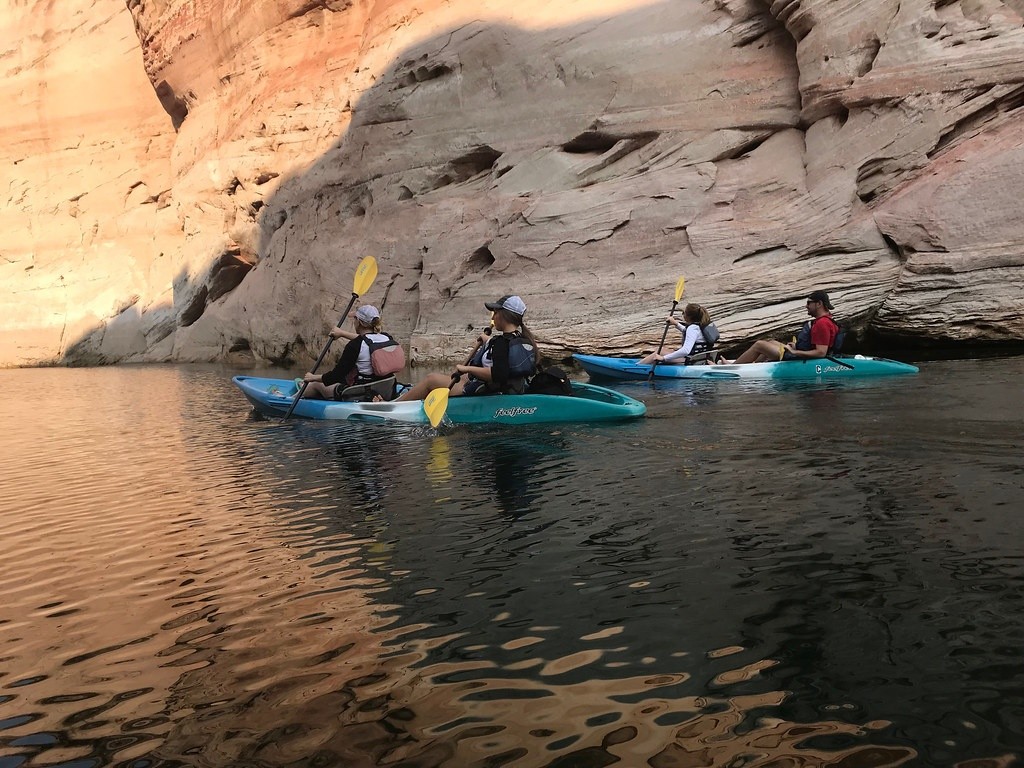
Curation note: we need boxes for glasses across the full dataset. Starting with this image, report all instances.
[807,301,817,305]
[682,311,687,316]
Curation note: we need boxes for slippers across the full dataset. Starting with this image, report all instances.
[372,395,386,402]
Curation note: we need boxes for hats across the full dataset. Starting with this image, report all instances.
[485,295,527,316]
[809,290,834,310]
[348,305,380,324]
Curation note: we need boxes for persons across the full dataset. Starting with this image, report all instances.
[634,304,710,364]
[707,290,838,364]
[371,295,539,401]
[267,305,395,400]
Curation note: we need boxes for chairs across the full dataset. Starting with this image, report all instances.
[341,373,396,402]
[684,350,719,365]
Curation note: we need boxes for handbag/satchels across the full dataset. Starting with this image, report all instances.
[531,366,575,396]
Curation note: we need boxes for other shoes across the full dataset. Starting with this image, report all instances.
[268,388,280,395]
[295,378,303,391]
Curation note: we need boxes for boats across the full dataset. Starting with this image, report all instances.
[571,353,921,381]
[231,375,649,425]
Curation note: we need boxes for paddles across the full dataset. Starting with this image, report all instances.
[648,276,685,382]
[779,336,797,361]
[284,255,378,421]
[423,310,497,428]
